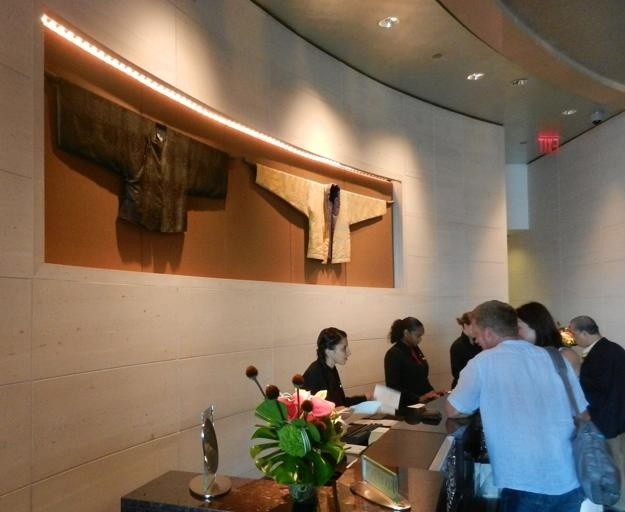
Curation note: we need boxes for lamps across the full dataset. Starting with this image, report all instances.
[469,72,482,80]
[380,17,398,28]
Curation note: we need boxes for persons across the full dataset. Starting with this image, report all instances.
[450,312,484,391]
[302,327,374,412]
[443,300,590,512]
[515,302,584,378]
[568,315,625,512]
[384,317,451,404]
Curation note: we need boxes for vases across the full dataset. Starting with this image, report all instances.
[289,462,315,503]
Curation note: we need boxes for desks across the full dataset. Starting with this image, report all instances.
[393,392,476,508]
[122,429,456,512]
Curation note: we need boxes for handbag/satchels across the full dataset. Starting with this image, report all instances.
[546,344,621,506]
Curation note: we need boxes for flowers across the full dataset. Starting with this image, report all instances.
[245,366,346,487]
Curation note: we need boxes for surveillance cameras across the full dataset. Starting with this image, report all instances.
[590,111,603,126]
